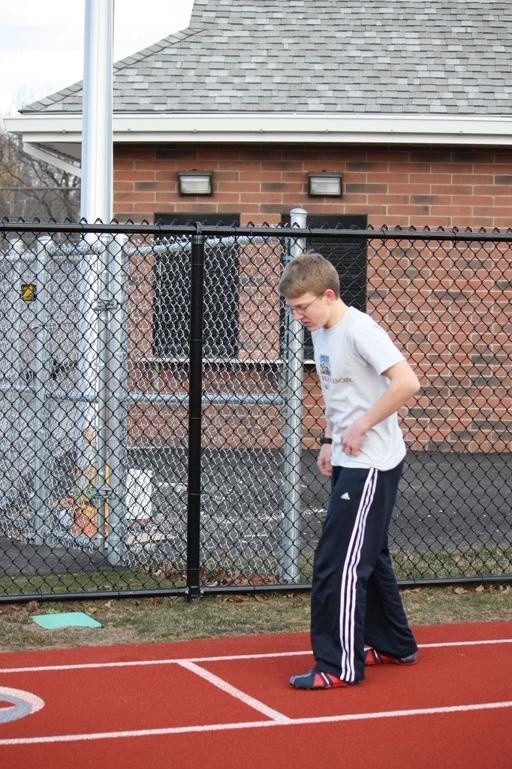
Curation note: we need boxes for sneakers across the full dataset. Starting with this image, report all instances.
[363,648,417,666]
[288,669,359,690]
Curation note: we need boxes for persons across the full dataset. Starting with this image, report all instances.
[277,248,422,692]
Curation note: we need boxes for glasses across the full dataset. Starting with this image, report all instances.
[285,296,319,315]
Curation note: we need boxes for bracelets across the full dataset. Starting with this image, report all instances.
[318,437,331,446]
